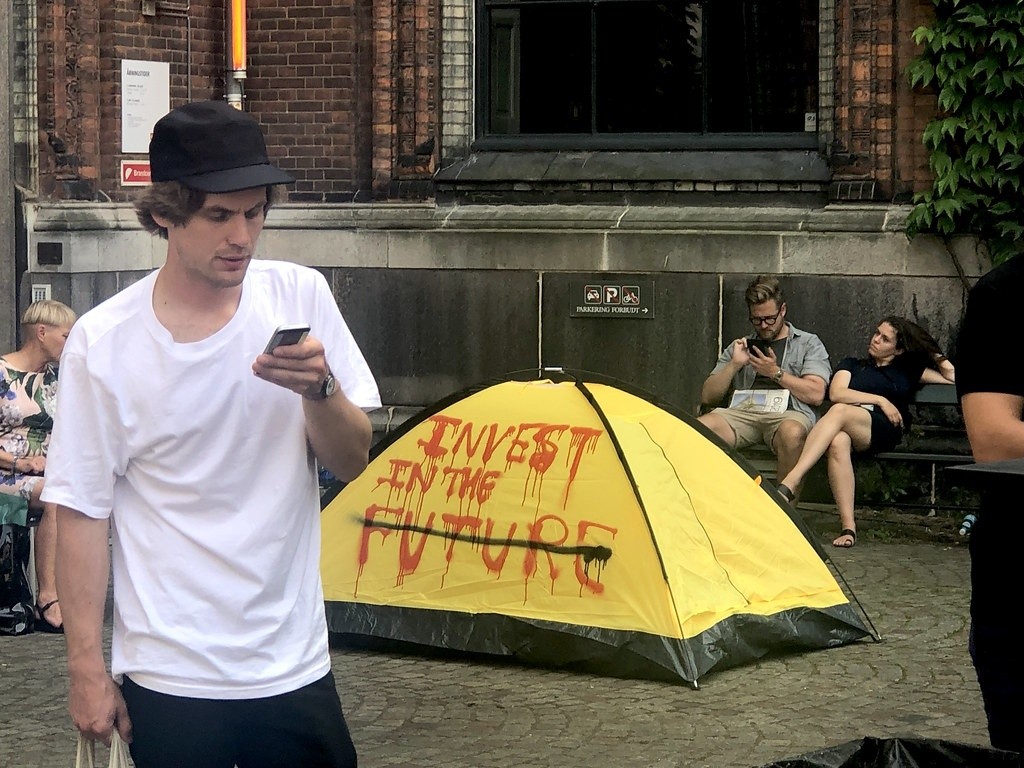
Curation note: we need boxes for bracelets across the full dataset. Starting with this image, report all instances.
[772,369,785,383]
[937,356,947,364]
[11,456,18,472]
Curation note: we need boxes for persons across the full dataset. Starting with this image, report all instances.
[697,278,832,508]
[776,316,956,547]
[0,300,75,635]
[38,101,381,768]
[950,251,1023,752]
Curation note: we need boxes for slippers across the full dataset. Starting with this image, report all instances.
[833,529,856,548]
[778,482,796,501]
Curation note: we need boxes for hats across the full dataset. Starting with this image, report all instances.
[149,100,296,194]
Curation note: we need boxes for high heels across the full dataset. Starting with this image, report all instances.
[34,599,63,633]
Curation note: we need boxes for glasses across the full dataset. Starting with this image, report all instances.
[749,308,781,325]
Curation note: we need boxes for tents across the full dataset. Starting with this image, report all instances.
[316,366,880,684]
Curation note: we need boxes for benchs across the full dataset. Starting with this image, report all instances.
[693,381,973,517]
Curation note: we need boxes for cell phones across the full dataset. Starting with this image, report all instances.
[746,338,766,359]
[253,322,311,376]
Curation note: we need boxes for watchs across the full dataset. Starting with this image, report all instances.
[301,371,335,400]
